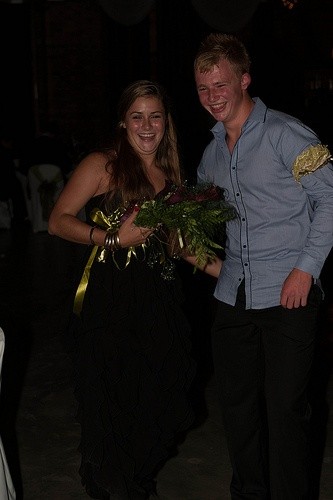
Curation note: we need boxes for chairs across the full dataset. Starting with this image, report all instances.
[0,164,86,232]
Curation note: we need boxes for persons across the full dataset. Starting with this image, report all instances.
[47,79,189,500]
[194,33,333,500]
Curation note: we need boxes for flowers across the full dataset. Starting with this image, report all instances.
[107,179,240,282]
